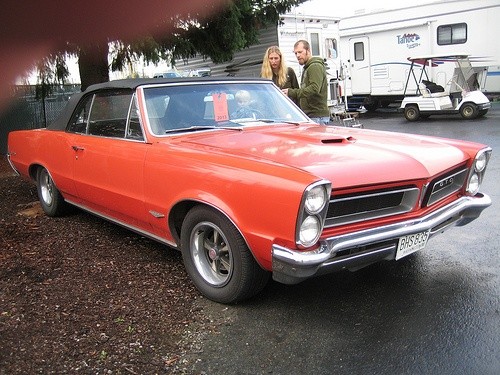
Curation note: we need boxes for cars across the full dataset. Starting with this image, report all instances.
[7,76,494,306]
[399,52,491,122]
[154,72,183,78]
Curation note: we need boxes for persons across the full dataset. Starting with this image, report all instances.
[230,90,262,125]
[257,45,301,115]
[279,38,331,126]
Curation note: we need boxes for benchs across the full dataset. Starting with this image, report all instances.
[419,82,450,98]
